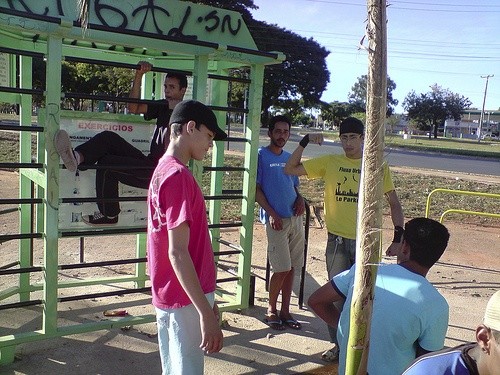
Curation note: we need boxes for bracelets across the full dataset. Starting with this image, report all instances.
[299,134,310,149]
[392,226,405,243]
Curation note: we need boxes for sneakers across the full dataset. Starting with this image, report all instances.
[82,211,119,227]
[54,129,77,172]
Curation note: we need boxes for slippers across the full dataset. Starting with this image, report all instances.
[285,314,302,329]
[263,317,285,330]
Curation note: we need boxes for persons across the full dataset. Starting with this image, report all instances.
[147,100,227,375]
[284,117,404,362]
[256,115,305,331]
[401,290,500,375]
[307,217,450,375]
[54,60,188,227]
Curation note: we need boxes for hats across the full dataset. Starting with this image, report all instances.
[168,99,227,141]
[483,290,499,331]
[338,117,364,135]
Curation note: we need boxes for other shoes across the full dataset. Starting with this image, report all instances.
[321,344,339,362]
